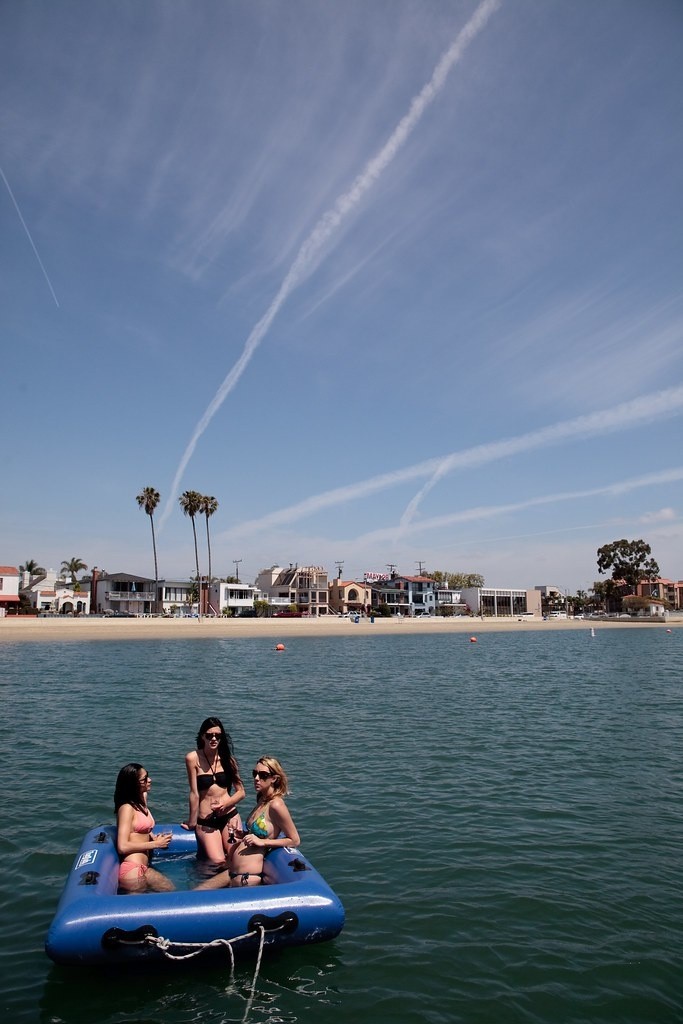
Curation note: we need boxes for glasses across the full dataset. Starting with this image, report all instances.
[139,771,149,784]
[204,733,222,740]
[252,769,272,779]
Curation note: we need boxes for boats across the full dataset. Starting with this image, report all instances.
[45,824,346,974]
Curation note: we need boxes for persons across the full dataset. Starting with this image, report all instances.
[190,755,300,891]
[180,716,246,875]
[114,762,176,894]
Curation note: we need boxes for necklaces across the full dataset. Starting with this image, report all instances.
[203,749,219,780]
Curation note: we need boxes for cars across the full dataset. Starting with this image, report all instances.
[185,613,223,618]
[517,611,633,619]
[415,611,431,618]
[271,609,302,618]
[230,610,258,618]
[342,611,359,618]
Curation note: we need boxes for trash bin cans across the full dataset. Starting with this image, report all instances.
[371,616,374,623]
[355,616,358,623]
